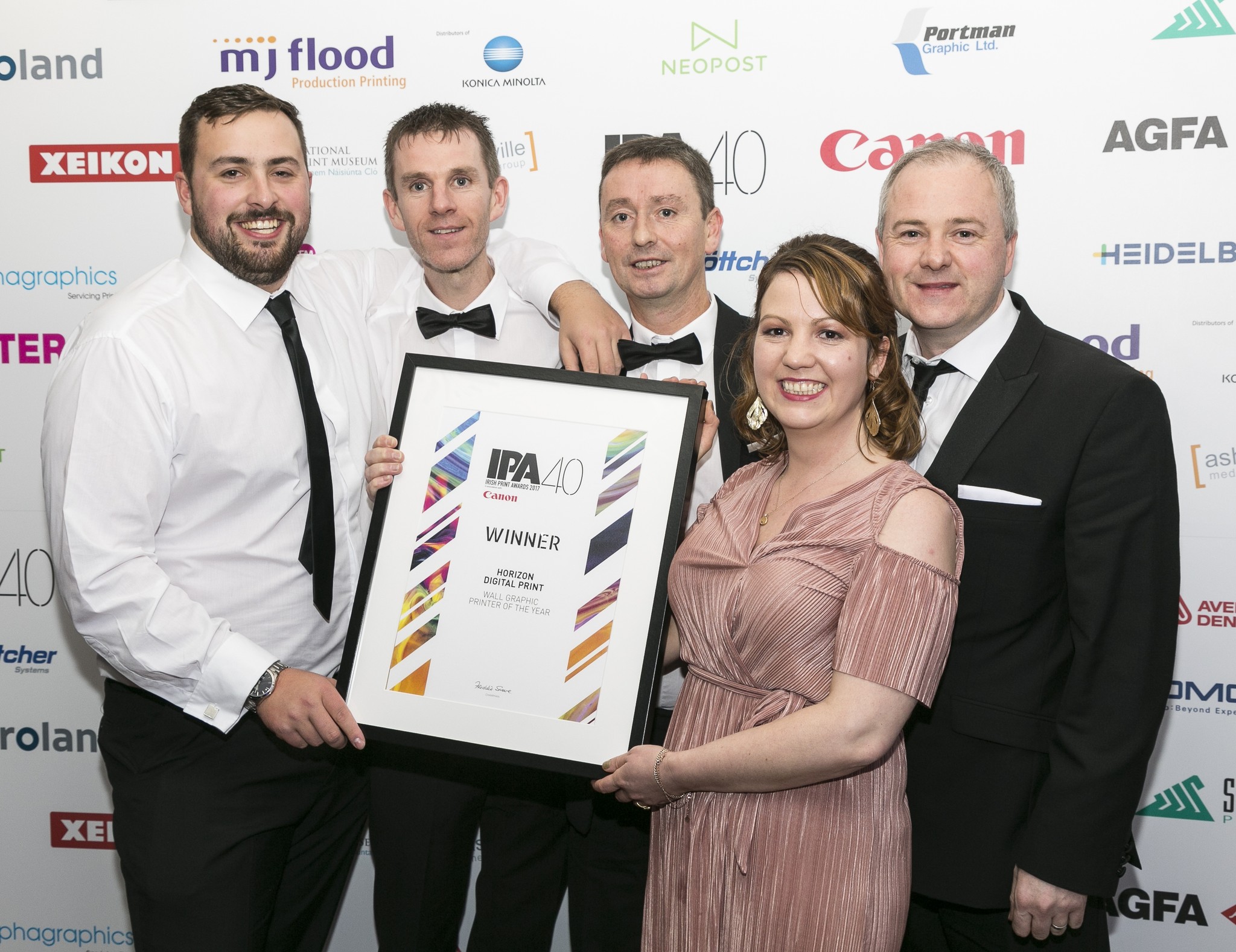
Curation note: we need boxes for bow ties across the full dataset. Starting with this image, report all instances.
[616,322,704,371]
[415,303,497,340]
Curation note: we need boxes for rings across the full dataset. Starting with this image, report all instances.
[1053,922,1068,930]
[636,802,651,810]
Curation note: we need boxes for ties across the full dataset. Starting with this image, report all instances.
[263,290,335,622]
[909,357,959,416]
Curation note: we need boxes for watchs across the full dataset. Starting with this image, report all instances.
[243,660,289,714]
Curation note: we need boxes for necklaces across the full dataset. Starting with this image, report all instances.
[760,441,870,528]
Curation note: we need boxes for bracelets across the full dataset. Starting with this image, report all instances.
[654,746,692,822]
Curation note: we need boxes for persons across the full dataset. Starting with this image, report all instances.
[364,139,783,952]
[292,102,566,952]
[43,82,633,952]
[638,232,961,952]
[875,143,1180,952]
